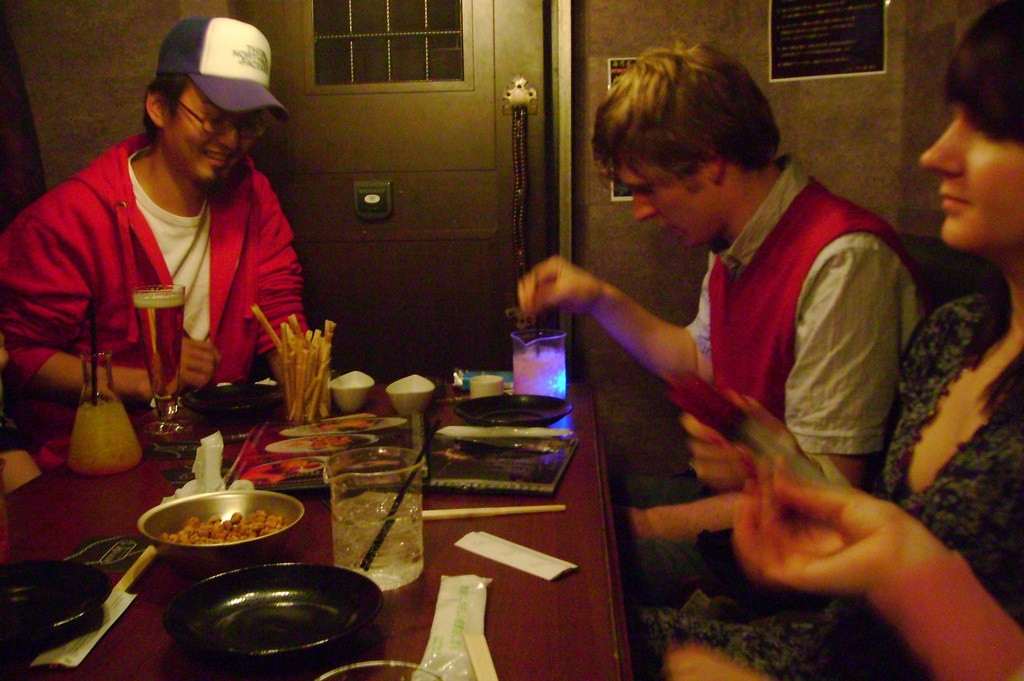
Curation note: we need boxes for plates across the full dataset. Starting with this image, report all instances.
[265,437,379,453]
[279,417,407,436]
[253,456,328,472]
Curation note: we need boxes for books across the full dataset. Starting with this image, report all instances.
[423,438,579,496]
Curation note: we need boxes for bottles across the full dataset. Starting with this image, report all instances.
[67,351,143,476]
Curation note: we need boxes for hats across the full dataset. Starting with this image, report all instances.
[156,15,291,124]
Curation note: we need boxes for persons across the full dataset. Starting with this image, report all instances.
[733,450,1024,681]
[0,14,308,480]
[513,39,925,540]
[625,0,1024,681]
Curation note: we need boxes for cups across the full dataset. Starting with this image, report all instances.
[324,446,424,591]
[510,329,566,399]
[133,285,191,435]
[314,661,445,681]
[276,355,331,424]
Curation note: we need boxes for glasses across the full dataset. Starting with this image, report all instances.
[177,98,266,142]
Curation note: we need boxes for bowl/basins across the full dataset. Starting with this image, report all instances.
[386,374,435,415]
[164,563,384,656]
[137,489,305,565]
[454,394,573,427]
[471,375,503,398]
[0,560,112,644]
[180,384,281,417]
[331,371,375,412]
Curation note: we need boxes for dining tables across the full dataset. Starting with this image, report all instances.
[0,374,637,681]
